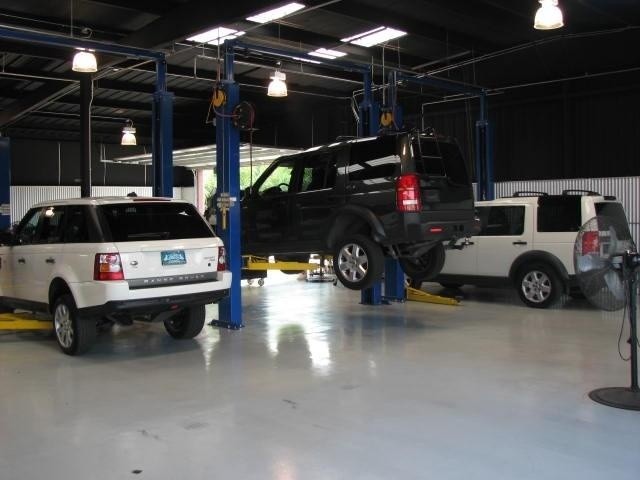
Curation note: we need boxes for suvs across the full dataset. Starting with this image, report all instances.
[0,197,233,355]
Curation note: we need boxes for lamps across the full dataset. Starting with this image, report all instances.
[266,71,288,98]
[71,51,98,73]
[533,0,565,30]
[120,127,137,146]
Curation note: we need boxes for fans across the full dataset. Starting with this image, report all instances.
[573,216,640,410]
